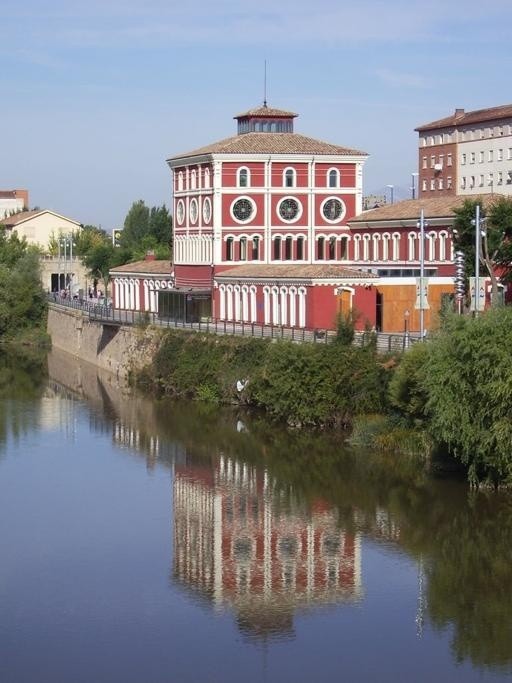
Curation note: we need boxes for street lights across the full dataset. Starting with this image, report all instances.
[410,558,427,643]
[58,239,64,296]
[469,217,484,321]
[64,238,69,298]
[409,170,419,198]
[416,219,429,340]
[70,238,76,301]
[386,184,394,204]
[56,400,79,444]
